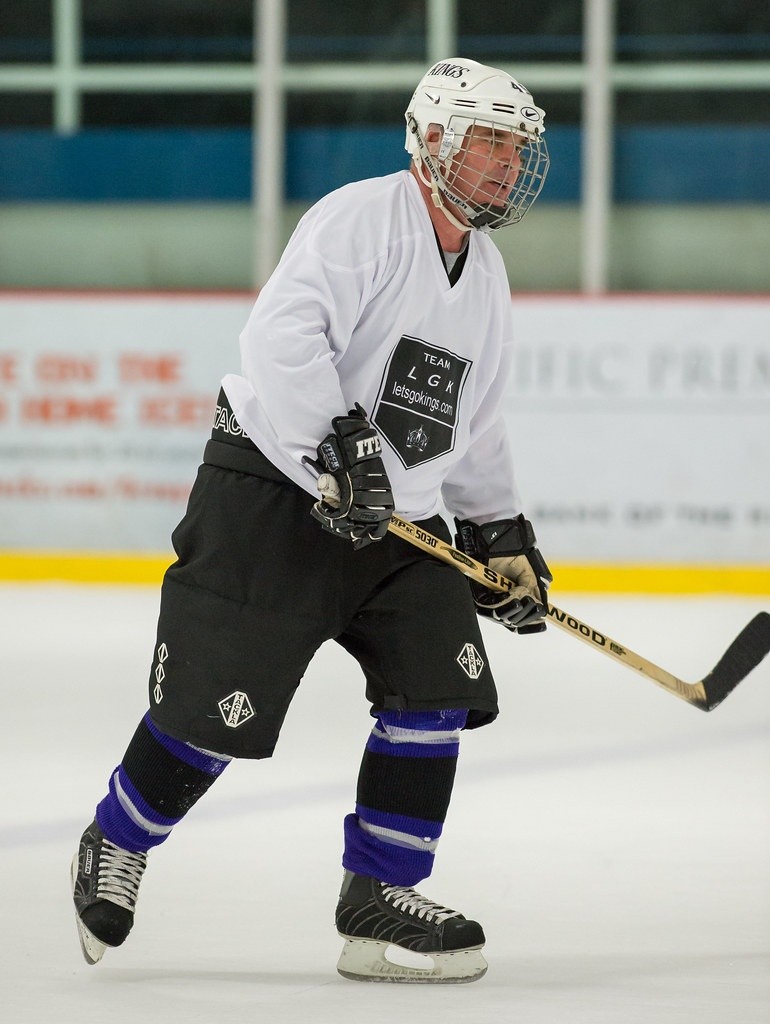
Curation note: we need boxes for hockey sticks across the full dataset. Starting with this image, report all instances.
[316,472,770,715]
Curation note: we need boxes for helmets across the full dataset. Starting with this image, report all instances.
[404,58,551,234]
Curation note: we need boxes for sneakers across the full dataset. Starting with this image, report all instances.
[68,819,147,965]
[336,869,487,983]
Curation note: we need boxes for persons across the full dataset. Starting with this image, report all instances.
[70,57,551,988]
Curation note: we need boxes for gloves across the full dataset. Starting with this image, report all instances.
[453,516,554,634]
[311,402,396,552]
